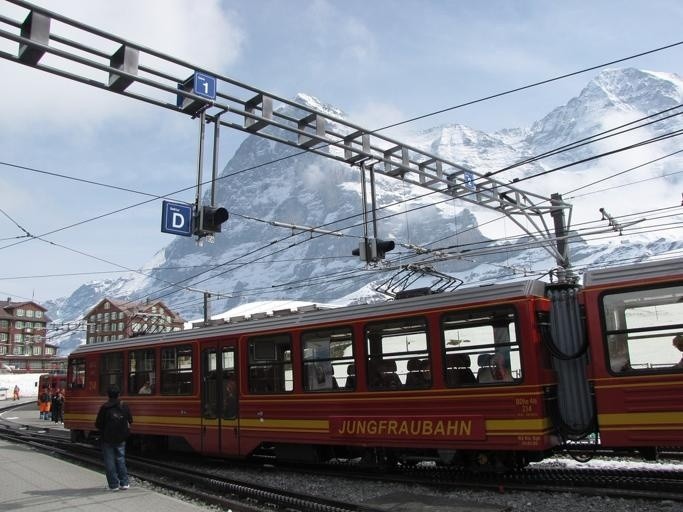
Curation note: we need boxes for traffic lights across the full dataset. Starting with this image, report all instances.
[204,204,229,233]
[372,238,394,260]
[351,240,369,261]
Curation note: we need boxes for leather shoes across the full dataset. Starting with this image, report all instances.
[50,419,64,425]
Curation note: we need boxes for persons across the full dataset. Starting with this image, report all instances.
[52,388,65,425]
[51,387,59,421]
[138,381,151,394]
[12,385,20,402]
[93,384,133,491]
[37,384,50,422]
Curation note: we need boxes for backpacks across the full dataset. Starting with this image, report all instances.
[39,393,49,403]
[105,400,130,443]
[54,395,63,406]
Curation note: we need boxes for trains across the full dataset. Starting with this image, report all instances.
[36,373,83,411]
[61,250,683,487]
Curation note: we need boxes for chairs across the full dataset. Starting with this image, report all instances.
[304,353,496,392]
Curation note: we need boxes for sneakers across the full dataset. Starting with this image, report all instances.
[13,398,19,401]
[40,416,49,420]
[105,481,119,492]
[118,483,130,490]
